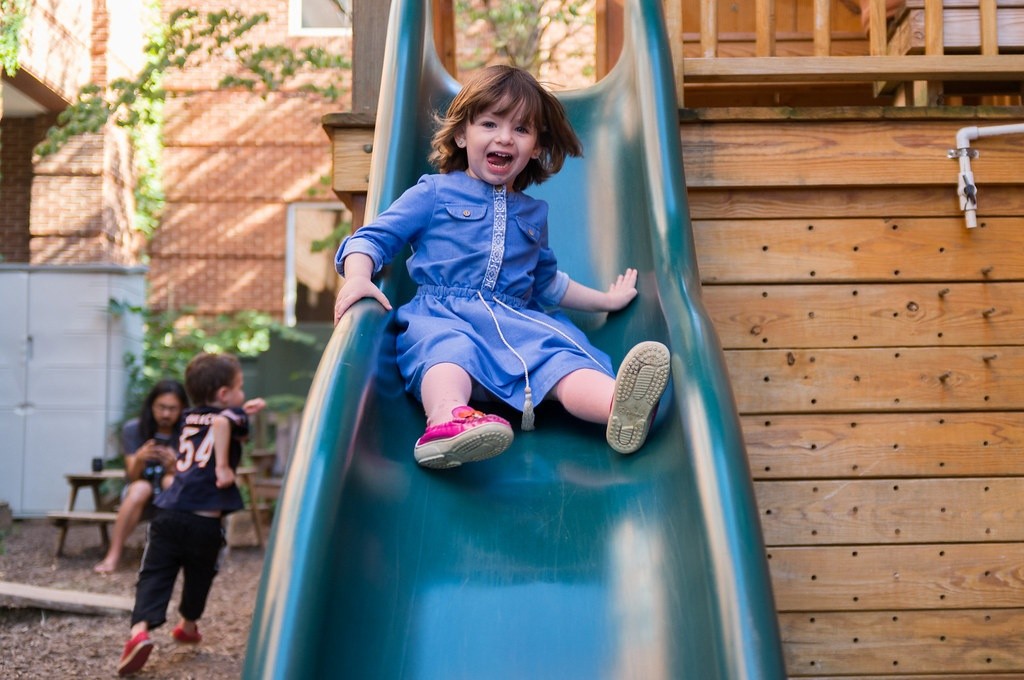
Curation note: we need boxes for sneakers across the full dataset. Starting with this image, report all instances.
[118,632,153,674]
[174,623,201,643]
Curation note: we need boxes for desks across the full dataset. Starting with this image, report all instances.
[48,465,267,559]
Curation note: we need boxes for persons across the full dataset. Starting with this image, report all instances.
[91,353,265,675]
[333,65,671,470]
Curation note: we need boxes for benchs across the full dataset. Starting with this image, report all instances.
[43,510,117,526]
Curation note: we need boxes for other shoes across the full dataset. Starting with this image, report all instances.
[414,405,514,469]
[606,341,670,454]
